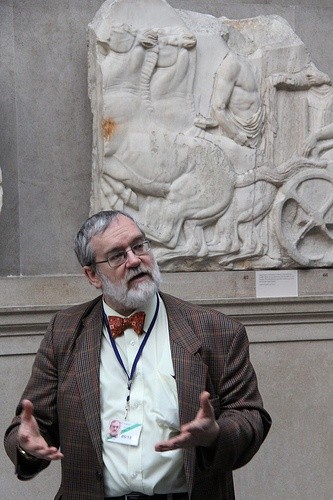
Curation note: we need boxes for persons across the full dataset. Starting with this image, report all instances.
[2,209,272,500]
[107,420,121,438]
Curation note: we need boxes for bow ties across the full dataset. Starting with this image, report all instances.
[108,311,146,340]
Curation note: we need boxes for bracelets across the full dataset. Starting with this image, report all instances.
[15,442,38,462]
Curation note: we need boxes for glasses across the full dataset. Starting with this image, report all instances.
[90,239,152,268]
